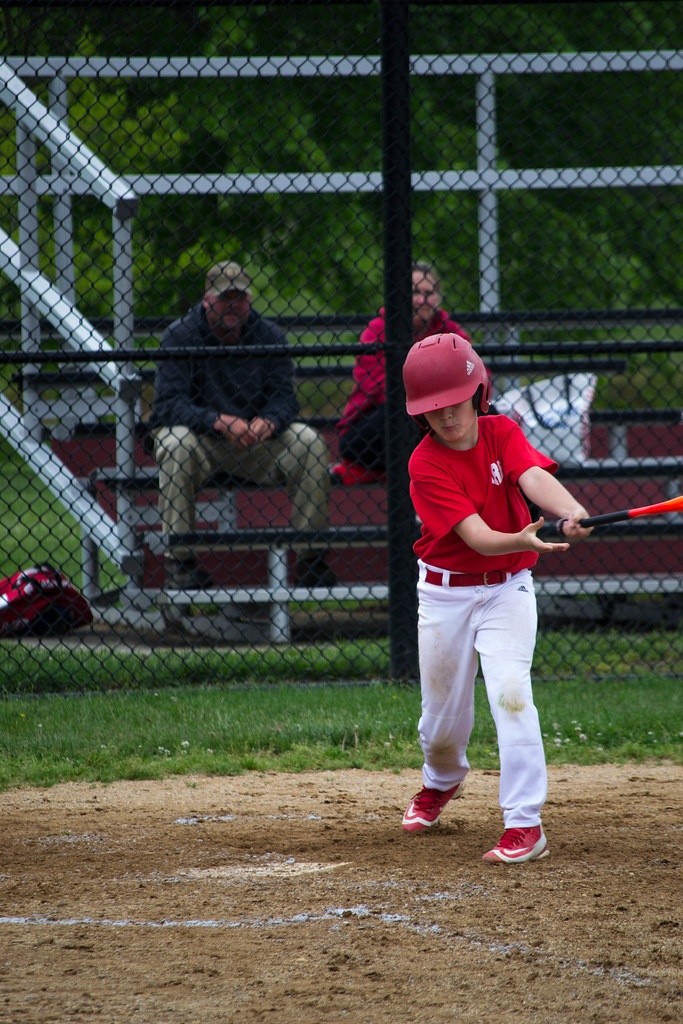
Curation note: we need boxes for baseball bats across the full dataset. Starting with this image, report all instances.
[556,493,683,538]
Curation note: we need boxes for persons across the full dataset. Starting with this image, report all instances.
[327,261,492,487]
[137,261,339,590]
[403,331,595,864]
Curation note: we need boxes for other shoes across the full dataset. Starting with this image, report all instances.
[163,555,213,589]
[294,557,339,587]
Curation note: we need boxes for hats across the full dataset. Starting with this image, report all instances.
[205,260,252,298]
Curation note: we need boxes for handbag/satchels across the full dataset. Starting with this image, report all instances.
[487,372,598,469]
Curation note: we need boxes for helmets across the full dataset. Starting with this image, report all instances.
[402,333,492,431]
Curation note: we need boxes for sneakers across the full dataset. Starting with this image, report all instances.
[481,824,551,864]
[402,781,466,833]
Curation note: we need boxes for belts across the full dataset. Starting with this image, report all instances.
[426,568,523,587]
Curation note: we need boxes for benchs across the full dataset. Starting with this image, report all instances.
[0,313,683,618]
